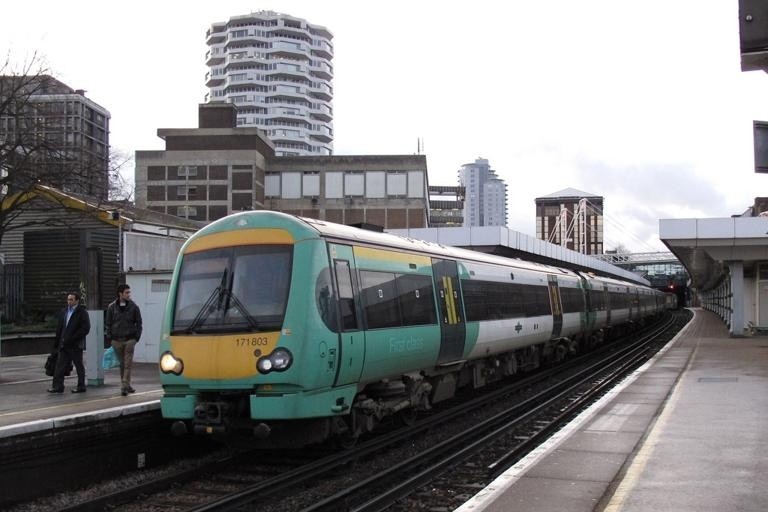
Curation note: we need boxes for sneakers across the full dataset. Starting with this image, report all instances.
[47,386,135,396]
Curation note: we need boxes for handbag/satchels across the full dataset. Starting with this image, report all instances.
[45,353,73,376]
[102,347,121,372]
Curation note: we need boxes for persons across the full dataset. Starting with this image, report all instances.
[105,284,142,397]
[47,291,90,393]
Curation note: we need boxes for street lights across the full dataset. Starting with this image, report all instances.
[109,210,124,287]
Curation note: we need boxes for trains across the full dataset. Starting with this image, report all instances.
[158,210,677,450]
